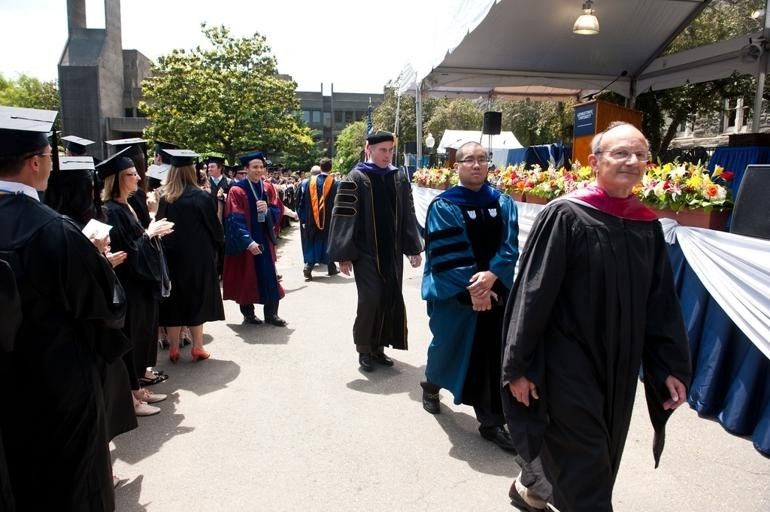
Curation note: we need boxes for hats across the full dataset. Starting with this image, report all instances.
[367,129,395,144]
[0,105,265,181]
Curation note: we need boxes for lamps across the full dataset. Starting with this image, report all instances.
[572,0,600,35]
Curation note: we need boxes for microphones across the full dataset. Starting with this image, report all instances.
[582,70,628,101]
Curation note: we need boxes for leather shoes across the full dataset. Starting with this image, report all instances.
[479,423,515,450]
[359,350,393,371]
[245,315,286,326]
[420,381,441,413]
[303,266,312,280]
[328,269,340,275]
[133,370,168,416]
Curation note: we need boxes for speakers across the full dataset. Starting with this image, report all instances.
[483,111,502,135]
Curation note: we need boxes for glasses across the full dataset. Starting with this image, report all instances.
[457,156,492,165]
[594,150,652,163]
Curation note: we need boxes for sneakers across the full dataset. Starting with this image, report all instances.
[509,481,554,511]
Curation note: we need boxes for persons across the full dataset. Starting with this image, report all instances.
[63,134,226,416]
[497,121,691,512]
[327,128,423,372]
[1,105,139,511]
[205,152,343,325]
[419,141,521,458]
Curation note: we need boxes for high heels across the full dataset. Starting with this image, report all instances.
[170,346,181,362]
[191,346,211,362]
